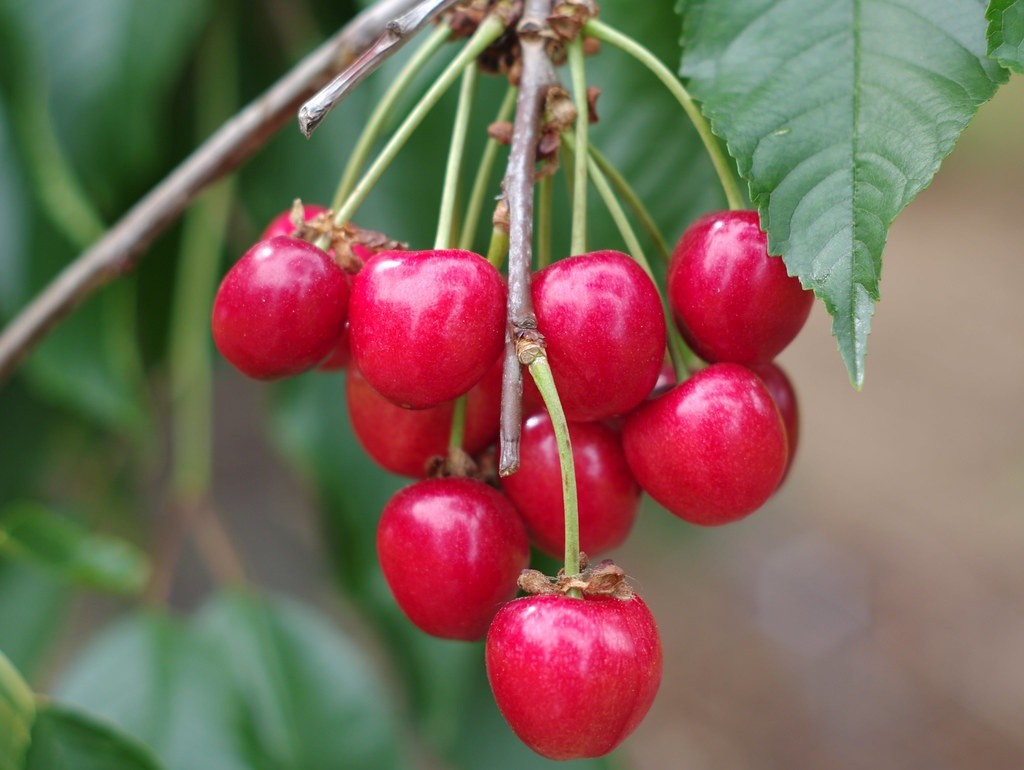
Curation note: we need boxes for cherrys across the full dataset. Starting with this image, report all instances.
[210,13,815,760]
